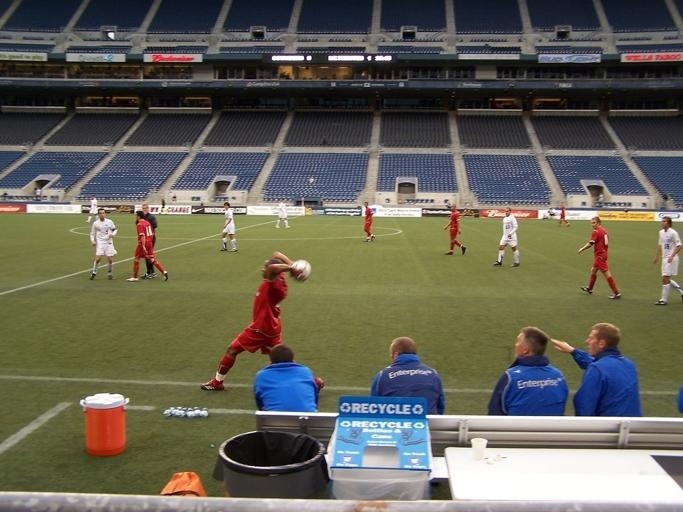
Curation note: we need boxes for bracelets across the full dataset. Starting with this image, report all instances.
[287,264,291,273]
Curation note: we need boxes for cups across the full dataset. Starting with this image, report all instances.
[469,438,489,459]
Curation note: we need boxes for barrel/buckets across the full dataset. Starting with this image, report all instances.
[79,393,130,455]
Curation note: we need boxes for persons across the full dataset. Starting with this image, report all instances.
[558,202,570,227]
[369,335,446,415]
[361,201,375,242]
[486,325,568,416]
[651,215,682,306]
[274,198,291,230]
[199,250,325,395]
[219,201,238,252]
[33,186,40,198]
[577,216,621,299]
[595,192,603,202]
[125,210,168,282]
[85,194,98,224]
[441,204,466,256]
[134,203,158,279]
[550,321,644,416]
[492,206,520,268]
[89,209,118,280]
[251,343,324,412]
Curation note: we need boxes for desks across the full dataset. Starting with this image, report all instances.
[438,444,681,509]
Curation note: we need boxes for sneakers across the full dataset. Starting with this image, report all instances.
[461,246,467,255]
[220,248,227,252]
[126,270,169,281]
[89,272,96,279]
[284,226,290,229]
[444,251,453,254]
[607,292,621,299]
[654,299,667,305]
[273,225,280,230]
[579,287,592,293]
[363,237,369,242]
[201,378,225,390]
[229,248,239,253]
[371,234,374,240]
[510,262,520,267]
[494,261,502,265]
[107,274,112,279]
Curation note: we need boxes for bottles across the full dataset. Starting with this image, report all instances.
[161,405,209,417]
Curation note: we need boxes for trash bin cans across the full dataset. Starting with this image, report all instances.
[213,430,329,498]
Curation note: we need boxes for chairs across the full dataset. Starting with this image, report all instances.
[0,1,682,65]
[0,101,683,215]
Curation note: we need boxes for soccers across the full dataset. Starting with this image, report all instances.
[291,259,311,281]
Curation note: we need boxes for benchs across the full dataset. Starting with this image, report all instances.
[248,404,681,458]
